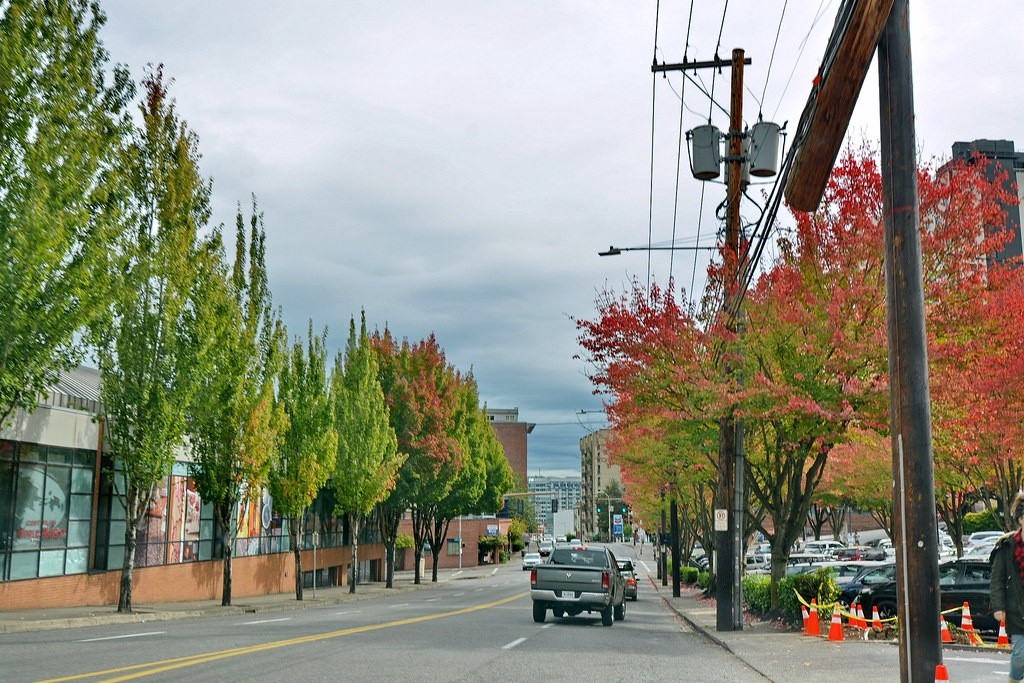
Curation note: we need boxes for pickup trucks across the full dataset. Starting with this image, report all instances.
[529,545,634,627]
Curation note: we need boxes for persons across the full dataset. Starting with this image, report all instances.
[591,554,606,566]
[853,530,861,546]
[990,490,1024,683]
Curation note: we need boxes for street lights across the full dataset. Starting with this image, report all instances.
[597,243,748,632]
[596,488,611,544]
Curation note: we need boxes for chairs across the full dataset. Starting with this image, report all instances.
[590,553,605,567]
[558,553,574,564]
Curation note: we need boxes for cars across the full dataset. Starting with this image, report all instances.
[567,538,584,546]
[555,534,567,542]
[521,553,543,570]
[680,529,1005,631]
[614,556,640,601]
[528,532,556,556]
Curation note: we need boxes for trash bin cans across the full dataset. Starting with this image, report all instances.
[522,551,527,557]
[525,542,529,546]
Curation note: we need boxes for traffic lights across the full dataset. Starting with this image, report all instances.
[597,503,602,513]
[621,503,627,513]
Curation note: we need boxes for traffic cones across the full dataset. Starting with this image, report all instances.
[802,597,824,636]
[800,605,810,631]
[961,601,982,646]
[940,614,958,644]
[871,605,885,639]
[823,605,847,641]
[857,604,868,629]
[934,663,951,683]
[847,603,858,627]
[995,621,1010,649]
